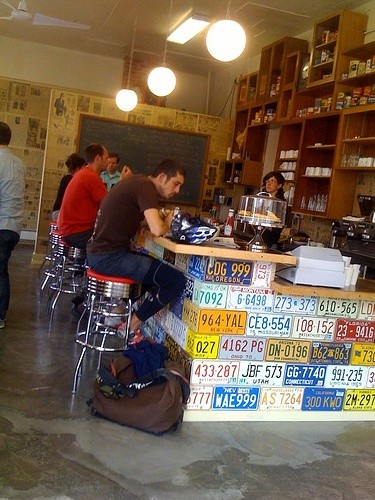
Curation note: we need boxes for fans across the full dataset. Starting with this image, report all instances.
[0,0,91,29]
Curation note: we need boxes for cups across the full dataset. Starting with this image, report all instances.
[358,157,375,168]
[278,149,299,180]
[305,166,331,177]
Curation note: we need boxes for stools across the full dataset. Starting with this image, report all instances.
[70,269,141,394]
[37,221,89,321]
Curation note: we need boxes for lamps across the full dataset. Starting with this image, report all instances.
[206,0,248,63]
[222,11,375,221]
[115,15,138,111]
[147,0,176,97]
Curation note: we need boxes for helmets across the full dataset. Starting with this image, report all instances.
[170,212,217,244]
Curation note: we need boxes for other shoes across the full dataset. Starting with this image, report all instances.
[0,318,7,329]
[71,296,86,313]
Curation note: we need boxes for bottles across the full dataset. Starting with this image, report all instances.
[223,209,235,237]
[300,194,328,213]
[340,153,359,167]
[211,206,218,222]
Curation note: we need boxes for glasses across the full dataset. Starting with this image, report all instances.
[265,179,276,184]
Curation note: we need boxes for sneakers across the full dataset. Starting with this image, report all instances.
[104,317,155,346]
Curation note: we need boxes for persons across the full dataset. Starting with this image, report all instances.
[101,154,120,193]
[120,156,150,181]
[54,92,65,116]
[234,170,292,247]
[57,143,110,303]
[87,158,187,345]
[51,153,87,221]
[0,121,24,328]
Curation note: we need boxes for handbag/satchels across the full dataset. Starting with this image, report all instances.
[93,354,189,434]
